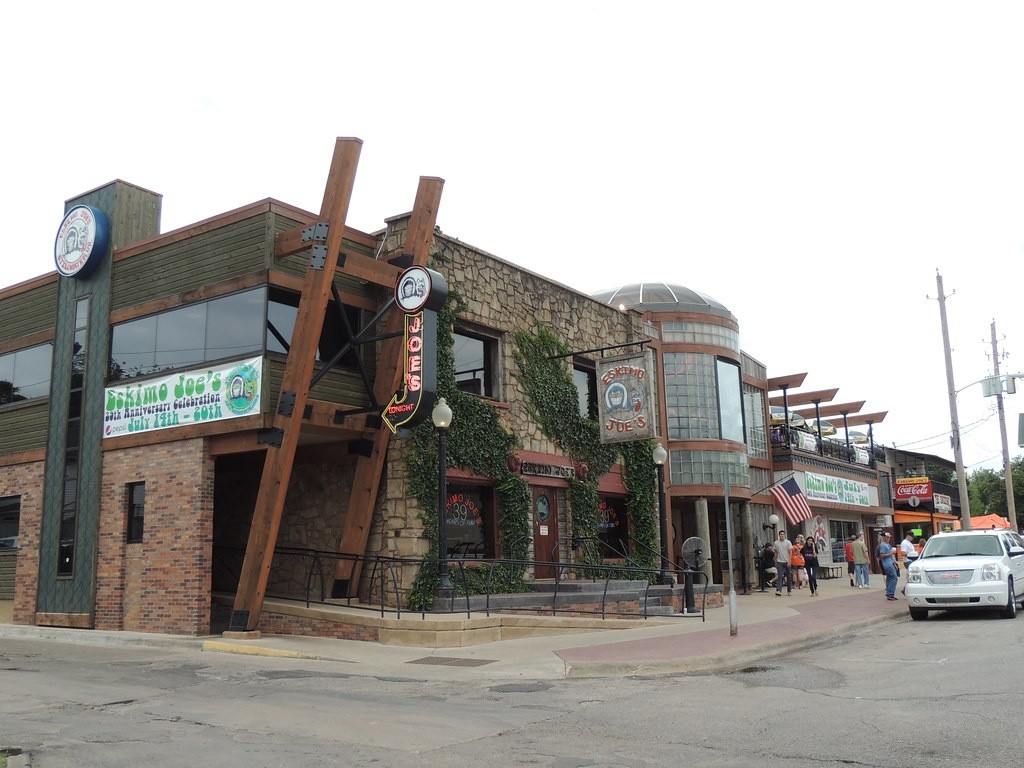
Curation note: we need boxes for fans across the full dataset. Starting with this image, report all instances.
[681,537,709,613]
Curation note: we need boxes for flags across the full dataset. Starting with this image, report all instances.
[768,476,814,528]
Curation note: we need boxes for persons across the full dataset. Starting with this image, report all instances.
[843,534,859,587]
[799,536,820,597]
[796,534,810,588]
[849,533,871,589]
[900,530,916,596]
[875,535,889,590]
[917,538,926,554]
[880,531,899,600]
[773,529,793,596]
[764,541,779,586]
[790,537,805,590]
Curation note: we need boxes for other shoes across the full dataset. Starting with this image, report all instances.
[859,585,862,589]
[892,596,898,600]
[815,591,819,596]
[787,592,791,596]
[775,592,783,597]
[887,598,894,600]
[850,578,854,586]
[810,593,815,597]
[864,584,871,589]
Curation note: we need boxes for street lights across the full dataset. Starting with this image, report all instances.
[652,442,674,584]
[432,397,455,597]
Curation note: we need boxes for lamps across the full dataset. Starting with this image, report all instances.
[763,513,779,530]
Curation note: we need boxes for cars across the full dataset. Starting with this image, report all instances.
[904,529,1024,620]
